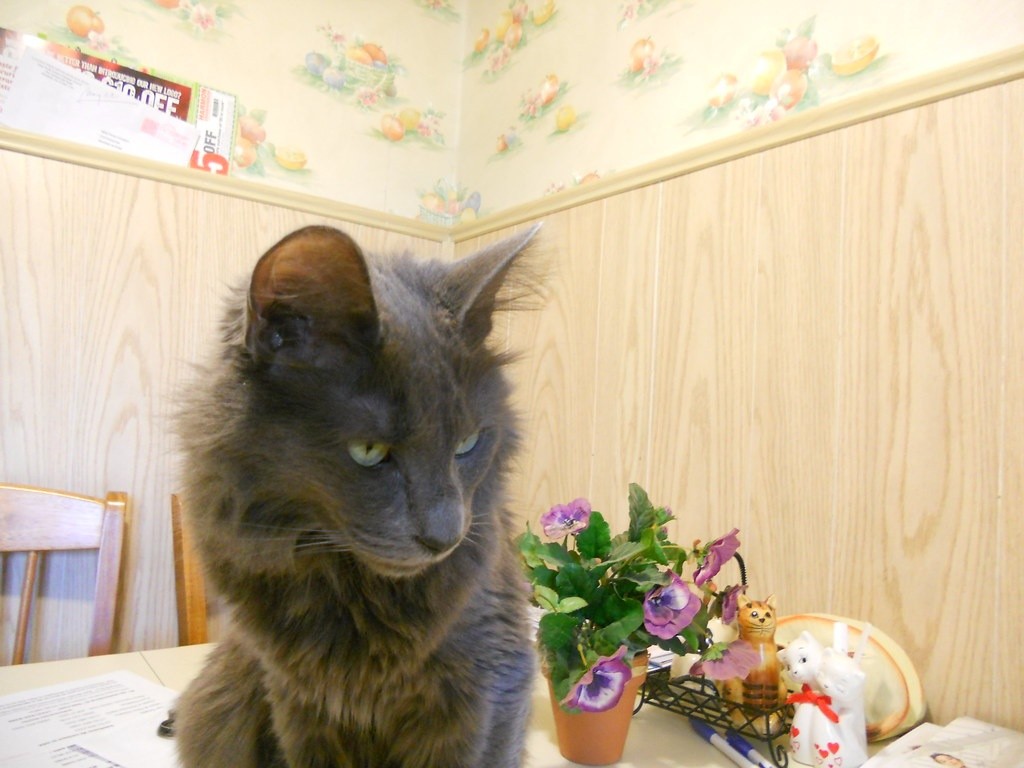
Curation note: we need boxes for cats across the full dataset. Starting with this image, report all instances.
[158,224,545,766]
[734,592,781,708]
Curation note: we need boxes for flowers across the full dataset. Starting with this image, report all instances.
[512,482,762,713]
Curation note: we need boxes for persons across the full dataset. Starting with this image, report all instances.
[931,752,967,768]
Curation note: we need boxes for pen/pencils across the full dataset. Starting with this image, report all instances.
[687,714,780,768]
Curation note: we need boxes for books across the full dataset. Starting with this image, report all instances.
[530,607,676,672]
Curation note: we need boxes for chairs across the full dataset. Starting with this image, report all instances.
[170,494,226,650]
[0,482,127,667]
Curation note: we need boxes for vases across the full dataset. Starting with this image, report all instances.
[541,647,648,765]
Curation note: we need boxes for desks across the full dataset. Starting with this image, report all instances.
[0,642,901,767]
